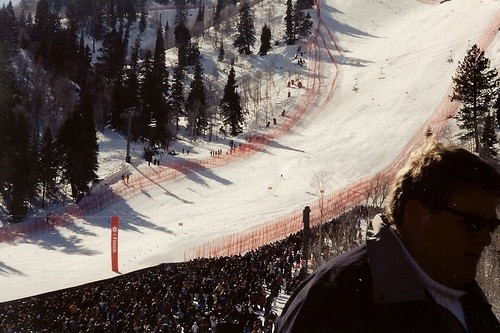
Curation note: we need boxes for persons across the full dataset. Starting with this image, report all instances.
[278,146,500,333]
[0,205,386,333]
[120,46,306,184]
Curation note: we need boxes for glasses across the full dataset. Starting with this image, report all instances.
[425,206,499,233]
